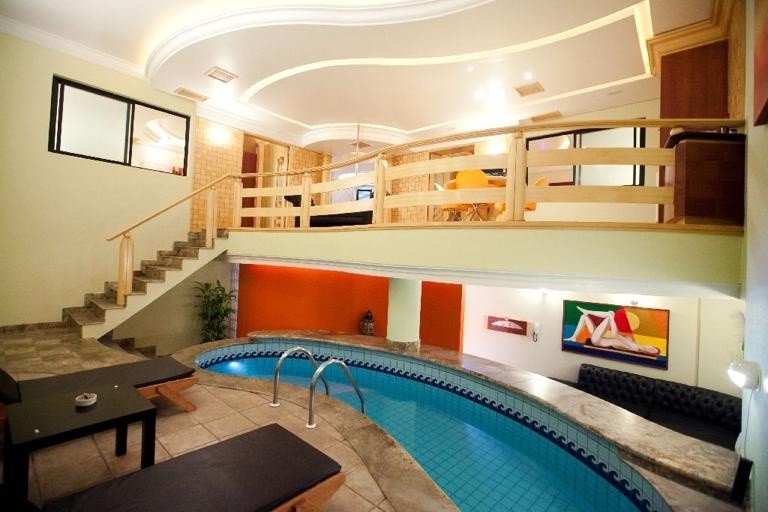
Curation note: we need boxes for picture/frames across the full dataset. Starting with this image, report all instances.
[561,298,671,372]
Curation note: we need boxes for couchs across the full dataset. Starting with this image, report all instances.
[550,363,741,451]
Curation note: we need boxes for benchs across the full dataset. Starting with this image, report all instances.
[54,354,200,413]
[40,420,348,512]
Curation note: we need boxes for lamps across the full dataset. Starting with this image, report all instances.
[727,358,761,395]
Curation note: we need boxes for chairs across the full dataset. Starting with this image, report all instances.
[432,168,549,221]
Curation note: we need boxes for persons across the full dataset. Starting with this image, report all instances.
[562,304,662,356]
